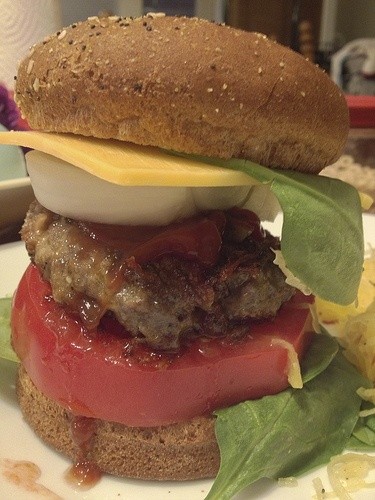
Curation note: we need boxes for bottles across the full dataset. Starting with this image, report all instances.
[325,95,375,204]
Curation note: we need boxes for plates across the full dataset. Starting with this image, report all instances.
[1,217,375,500]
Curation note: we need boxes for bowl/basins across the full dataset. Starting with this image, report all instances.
[0,176,32,234]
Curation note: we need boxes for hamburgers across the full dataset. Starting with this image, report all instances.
[0,14,375,493]
